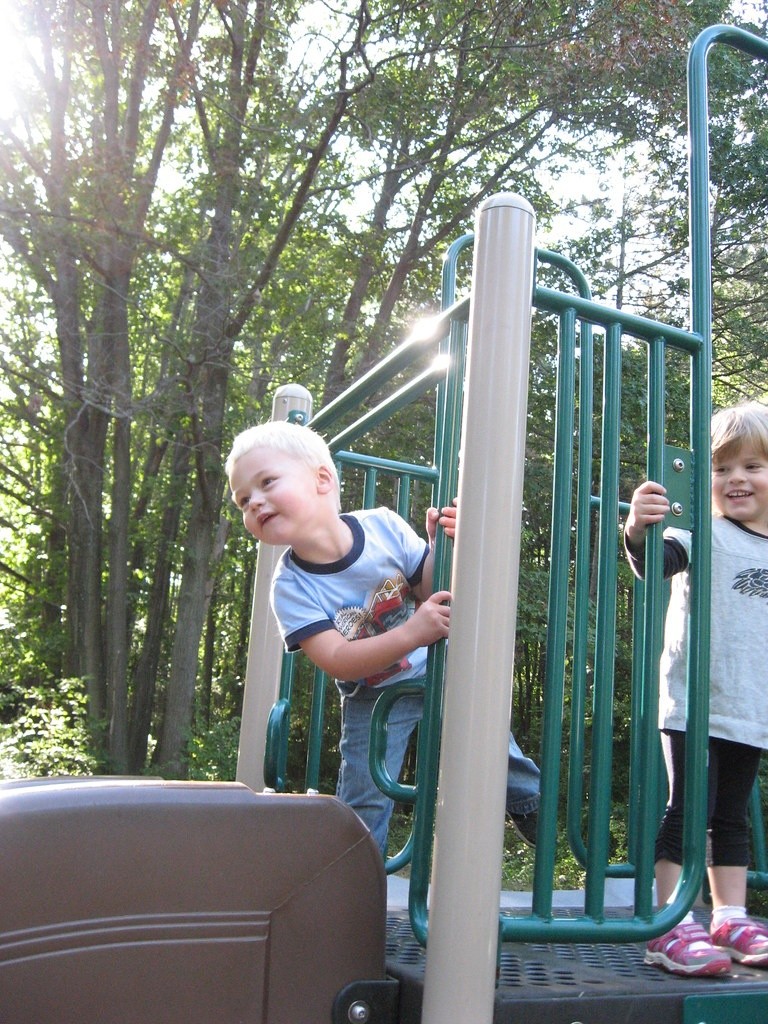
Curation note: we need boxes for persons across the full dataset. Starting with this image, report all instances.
[622,400,768,978]
[225,420,540,875]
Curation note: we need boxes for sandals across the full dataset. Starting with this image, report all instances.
[643,921,731,977]
[710,917,768,968]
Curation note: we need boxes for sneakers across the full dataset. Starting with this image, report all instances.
[506,808,537,849]
